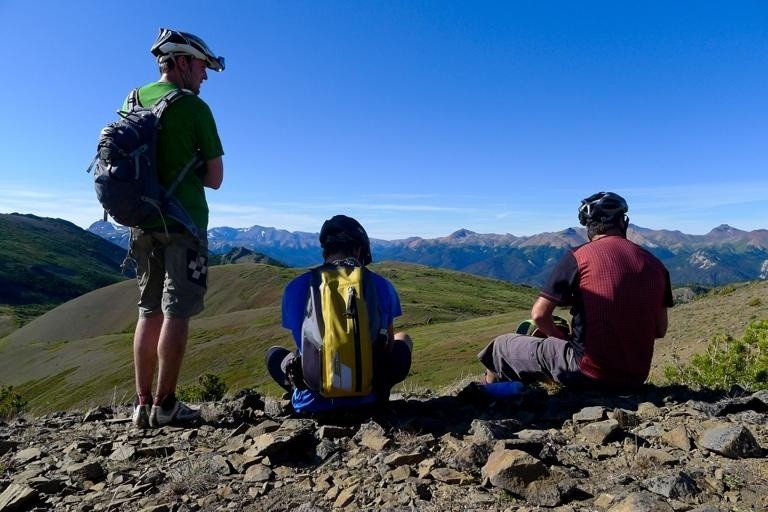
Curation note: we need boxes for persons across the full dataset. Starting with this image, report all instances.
[120,28,223,429]
[476,192,673,395]
[265,215,413,424]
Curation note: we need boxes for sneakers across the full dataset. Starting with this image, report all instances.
[131,401,200,428]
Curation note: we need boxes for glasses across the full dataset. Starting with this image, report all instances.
[319,215,372,264]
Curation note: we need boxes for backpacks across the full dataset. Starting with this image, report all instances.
[93,88,197,228]
[302,266,373,398]
[515,315,570,337]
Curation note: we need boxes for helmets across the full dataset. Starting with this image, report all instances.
[151,28,224,71]
[578,191,628,225]
